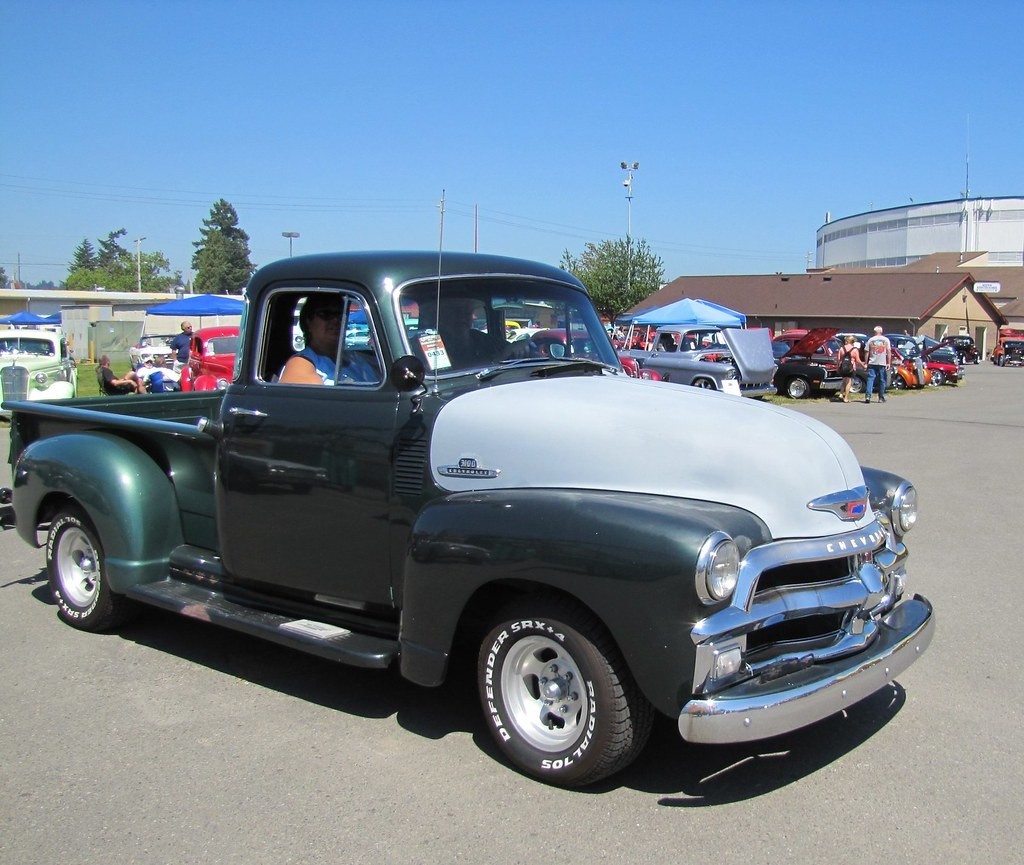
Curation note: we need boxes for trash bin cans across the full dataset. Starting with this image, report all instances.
[0,367,32,403]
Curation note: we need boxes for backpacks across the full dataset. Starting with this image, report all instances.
[838,345,857,377]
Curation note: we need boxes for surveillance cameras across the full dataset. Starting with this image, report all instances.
[623,180,629,186]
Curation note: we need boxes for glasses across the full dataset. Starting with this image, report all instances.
[306,309,344,321]
[186,325,192,329]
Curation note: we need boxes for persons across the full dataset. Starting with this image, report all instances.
[96,355,167,395]
[277,293,383,385]
[836,325,911,404]
[170,321,196,373]
[941,333,949,342]
[409,294,538,369]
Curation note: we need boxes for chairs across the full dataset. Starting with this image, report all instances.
[96,366,111,397]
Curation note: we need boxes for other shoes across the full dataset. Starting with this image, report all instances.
[878,396,887,403]
[839,394,843,398]
[844,395,851,402]
[864,397,871,403]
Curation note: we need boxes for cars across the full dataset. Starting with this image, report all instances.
[525,328,661,382]
[0,327,241,414]
[746,327,1024,399]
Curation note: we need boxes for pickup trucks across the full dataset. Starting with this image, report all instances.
[1,249,936,788]
[617,325,778,397]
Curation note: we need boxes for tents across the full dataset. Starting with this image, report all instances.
[611,297,748,351]
[146,292,246,329]
[0,310,61,324]
